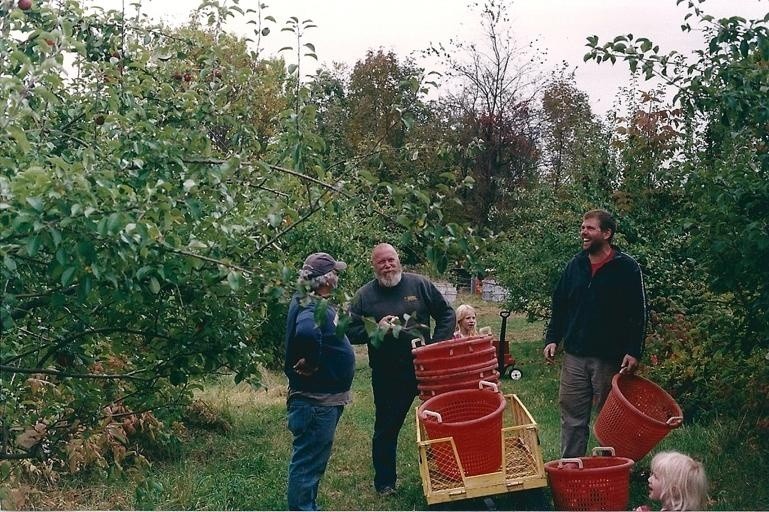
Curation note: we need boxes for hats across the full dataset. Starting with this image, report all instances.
[303,252,348,281]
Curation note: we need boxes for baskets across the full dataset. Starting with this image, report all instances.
[411,326,507,483]
[593,367,683,463]
[544,446,635,510]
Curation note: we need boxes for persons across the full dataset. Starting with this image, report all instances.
[452,304,480,338]
[343,243,457,495]
[543,209,648,458]
[630,451,707,512]
[283,252,357,510]
